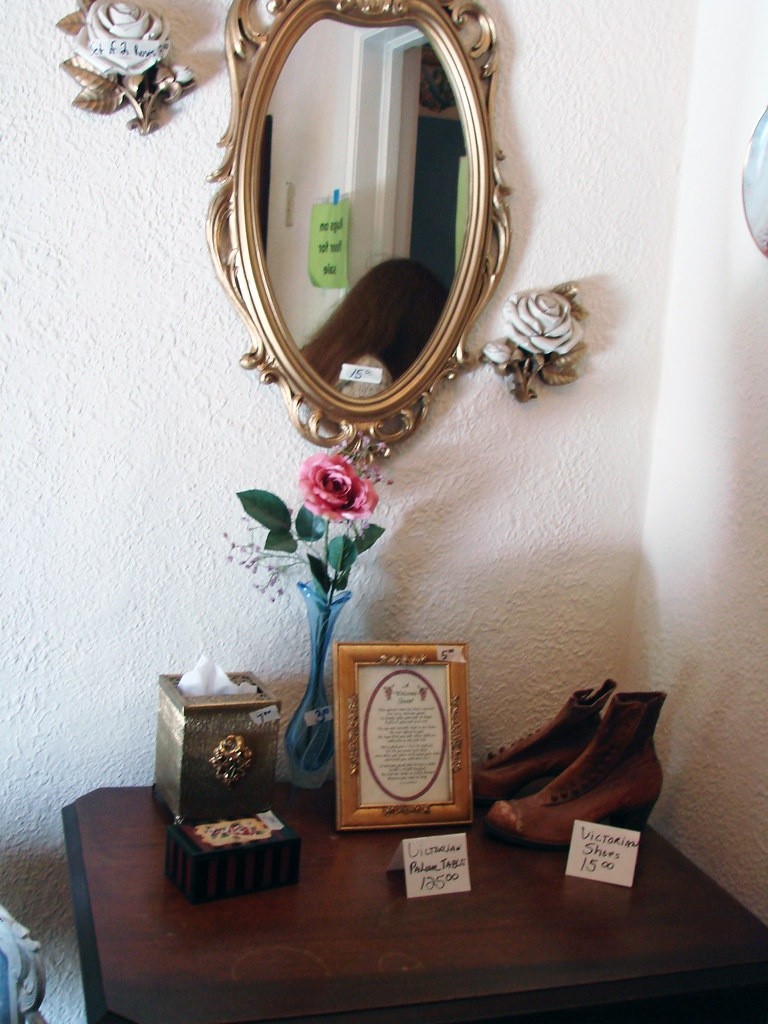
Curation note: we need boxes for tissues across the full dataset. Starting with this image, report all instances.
[152,654,280,819]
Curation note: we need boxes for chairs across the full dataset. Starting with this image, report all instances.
[0,906,48,1024]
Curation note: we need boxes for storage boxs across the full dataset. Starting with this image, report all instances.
[163,811,300,906]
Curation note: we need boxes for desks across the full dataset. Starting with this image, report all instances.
[62,773,768,1024]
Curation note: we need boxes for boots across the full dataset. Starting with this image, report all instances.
[483,690,666,848]
[471,678,617,806]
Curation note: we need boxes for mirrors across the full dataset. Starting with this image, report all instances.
[210,0,506,459]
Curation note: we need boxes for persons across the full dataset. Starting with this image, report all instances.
[298,259,445,400]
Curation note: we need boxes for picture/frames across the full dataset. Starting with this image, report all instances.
[334,641,474,831]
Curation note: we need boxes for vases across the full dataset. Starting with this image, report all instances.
[275,583,351,788]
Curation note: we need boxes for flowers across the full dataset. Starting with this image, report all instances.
[232,453,394,602]
[481,283,586,402]
[57,0,196,136]
